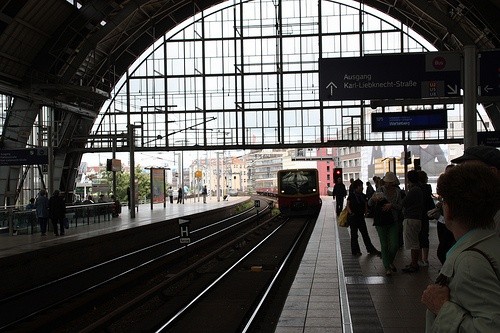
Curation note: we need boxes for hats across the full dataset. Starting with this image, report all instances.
[383,172,396,182]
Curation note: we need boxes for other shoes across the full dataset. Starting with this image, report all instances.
[370,249,381,255]
[386,270,391,277]
[424,258,429,264]
[401,263,419,273]
[354,252,362,255]
[390,262,397,272]
[419,257,424,264]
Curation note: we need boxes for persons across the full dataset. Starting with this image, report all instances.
[347,178,379,256]
[451,146,500,231]
[366,181,374,199]
[88,196,95,203]
[167,187,173,203]
[36,189,50,235]
[98,194,108,203]
[435,164,454,266]
[177,188,182,204]
[202,185,206,203]
[224,185,232,199]
[333,146,500,333]
[416,169,435,266]
[50,190,66,232]
[384,169,421,269]
[420,160,500,333]
[25,198,36,209]
[333,178,347,216]
[369,171,403,274]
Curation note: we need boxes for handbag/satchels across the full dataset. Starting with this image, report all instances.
[337,206,350,227]
[372,187,395,227]
[64,217,69,229]
